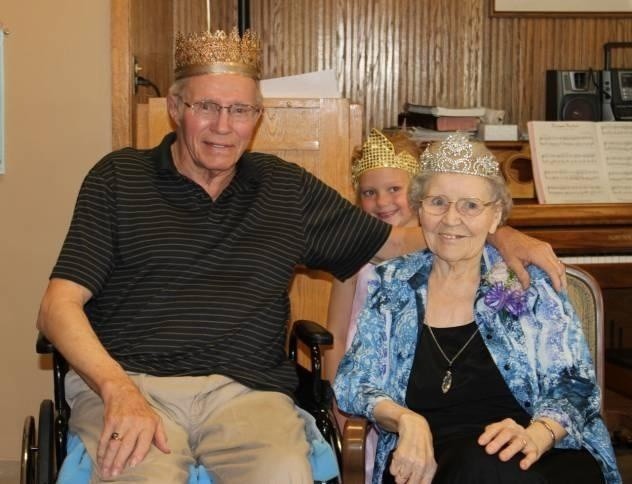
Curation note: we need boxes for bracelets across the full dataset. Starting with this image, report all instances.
[533,418,557,450]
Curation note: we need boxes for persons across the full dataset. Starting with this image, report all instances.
[322,126,422,443]
[330,123,625,484]
[35,25,568,484]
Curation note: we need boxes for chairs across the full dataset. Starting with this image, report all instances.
[341,262,606,484]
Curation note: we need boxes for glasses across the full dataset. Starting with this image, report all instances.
[176,94,260,119]
[419,195,494,218]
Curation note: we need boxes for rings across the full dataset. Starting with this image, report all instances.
[109,431,123,442]
[520,439,528,446]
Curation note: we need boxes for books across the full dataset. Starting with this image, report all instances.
[395,102,485,141]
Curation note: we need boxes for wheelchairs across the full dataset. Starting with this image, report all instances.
[20,319,343,484]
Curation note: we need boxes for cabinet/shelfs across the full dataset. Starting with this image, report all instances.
[135,97,364,381]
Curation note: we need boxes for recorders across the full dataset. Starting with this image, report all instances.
[545,41,632,121]
